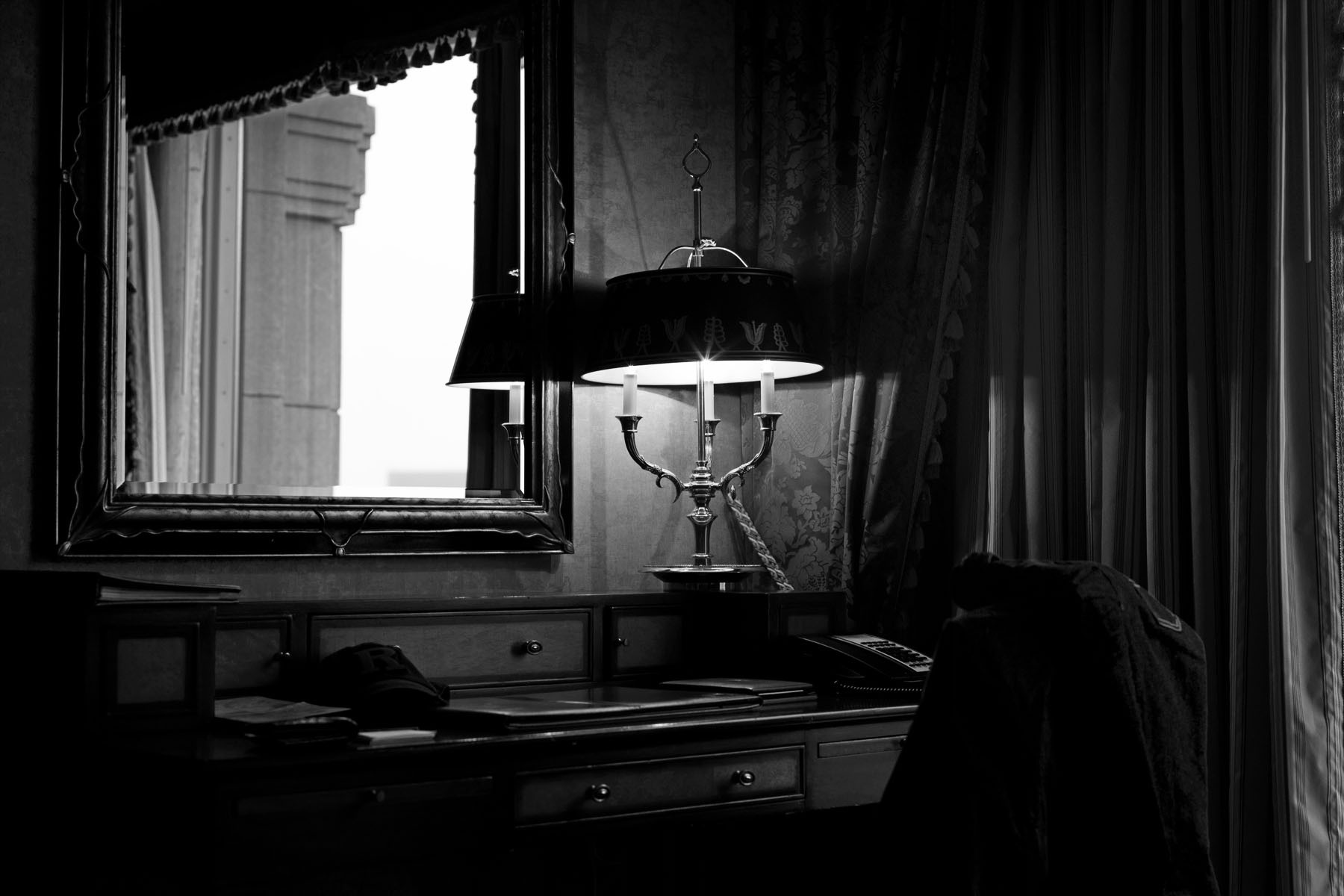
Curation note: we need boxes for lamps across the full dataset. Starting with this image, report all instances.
[578,138,832,589]
[449,292,540,498]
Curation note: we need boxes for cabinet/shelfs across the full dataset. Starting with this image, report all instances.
[63,594,919,896]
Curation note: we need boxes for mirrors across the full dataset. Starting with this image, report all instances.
[27,0,572,562]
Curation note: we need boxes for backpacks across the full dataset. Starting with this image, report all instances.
[875,552,1218,895]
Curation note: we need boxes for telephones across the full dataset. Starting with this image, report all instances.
[783,632,933,691]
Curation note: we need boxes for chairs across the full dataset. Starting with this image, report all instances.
[684,552,1220,896]
